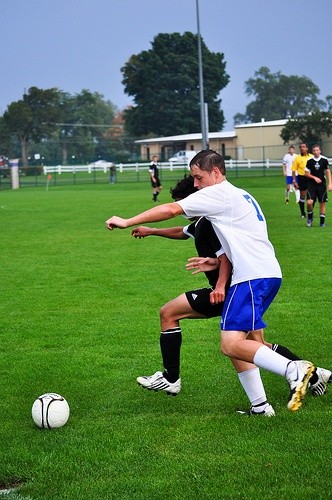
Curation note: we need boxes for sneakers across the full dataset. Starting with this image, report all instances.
[310,367,331,396]
[285,360,314,413]
[320,224,326,227]
[307,219,313,227]
[237,402,275,418]
[302,213,305,218]
[285,198,289,204]
[137,369,182,396]
[152,198,160,202]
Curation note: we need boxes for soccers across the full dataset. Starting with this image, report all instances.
[31,393,70,429]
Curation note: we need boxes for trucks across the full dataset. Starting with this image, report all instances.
[167,150,196,163]
